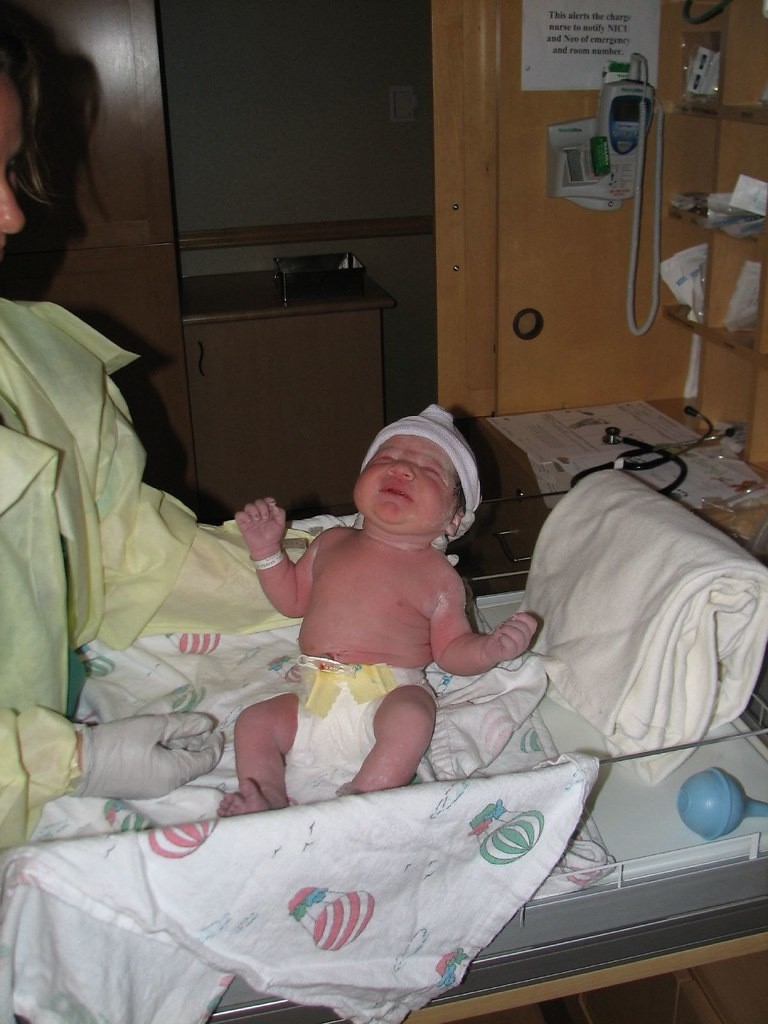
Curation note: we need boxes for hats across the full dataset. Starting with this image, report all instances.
[360,404,482,544]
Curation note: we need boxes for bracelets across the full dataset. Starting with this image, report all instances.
[249,548,286,570]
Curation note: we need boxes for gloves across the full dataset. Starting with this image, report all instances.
[69,712,224,799]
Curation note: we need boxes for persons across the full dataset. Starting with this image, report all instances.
[0,35,478,854]
[217,404,539,818]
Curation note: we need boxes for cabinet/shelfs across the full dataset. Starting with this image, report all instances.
[182,256,395,518]
[0,0,196,512]
[661,1,768,465]
